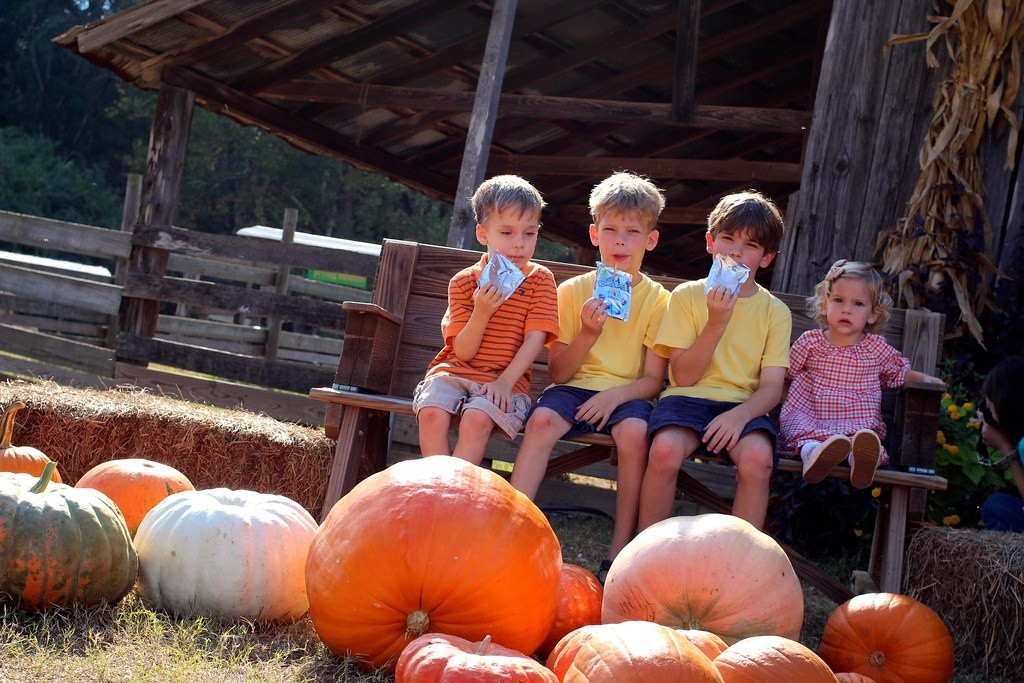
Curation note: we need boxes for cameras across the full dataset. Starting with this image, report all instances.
[973,397,998,427]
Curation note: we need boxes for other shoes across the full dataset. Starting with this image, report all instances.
[801,434,852,484]
[848,429,881,489]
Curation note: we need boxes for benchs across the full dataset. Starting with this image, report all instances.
[309,238,949,607]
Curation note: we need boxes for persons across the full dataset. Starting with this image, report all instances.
[781,260,945,488]
[636,191,793,532]
[979,356,1024,534]
[510,173,671,584]
[412,174,560,466]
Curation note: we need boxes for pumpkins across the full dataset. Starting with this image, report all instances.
[303,455,955,683]
[0,401,320,624]
